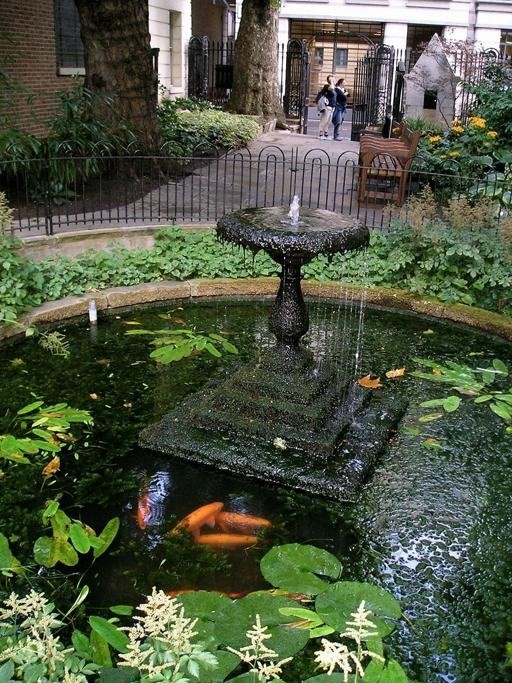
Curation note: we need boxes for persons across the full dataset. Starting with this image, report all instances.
[332,79,351,141]
[315,75,337,140]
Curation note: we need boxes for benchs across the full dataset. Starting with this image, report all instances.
[359,118,423,206]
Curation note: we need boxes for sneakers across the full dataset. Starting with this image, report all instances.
[320,135,343,141]
